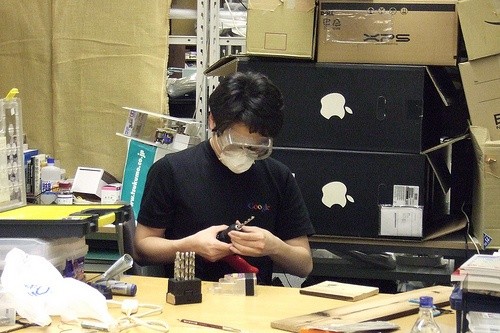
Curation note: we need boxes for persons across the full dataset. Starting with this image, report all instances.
[133,71,314,287]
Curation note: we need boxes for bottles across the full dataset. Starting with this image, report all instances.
[411,296,441,333]
[41,158,60,194]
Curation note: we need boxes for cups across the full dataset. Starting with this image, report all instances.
[0,293,16,326]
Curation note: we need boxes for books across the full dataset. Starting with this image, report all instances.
[24,154,46,195]
[451,253,500,292]
[300,281,380,302]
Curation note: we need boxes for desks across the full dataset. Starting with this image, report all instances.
[308,231,482,250]
[0,198,125,276]
[0,274,460,333]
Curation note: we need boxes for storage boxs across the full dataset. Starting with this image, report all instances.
[245,1,317,60]
[458,55,500,140]
[455,0,500,61]
[101,183,121,205]
[465,118,500,250]
[70,167,119,199]
[316,2,459,66]
